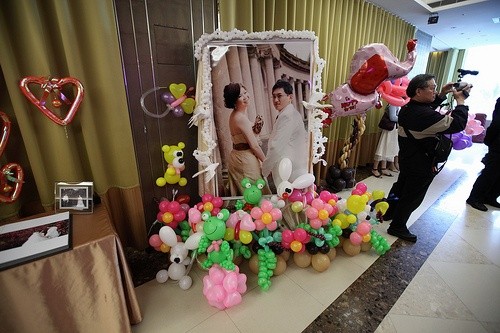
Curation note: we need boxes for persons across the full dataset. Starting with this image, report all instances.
[223,82,273,197]
[62,194,69,207]
[370,104,401,178]
[382,74,470,243]
[76,195,85,208]
[262,78,309,188]
[466,97,500,211]
[22,225,61,247]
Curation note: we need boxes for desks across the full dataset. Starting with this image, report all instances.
[0,200,142,333]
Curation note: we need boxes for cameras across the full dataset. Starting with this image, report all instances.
[454,81,468,92]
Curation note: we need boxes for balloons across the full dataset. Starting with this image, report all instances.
[156,142,187,186]
[435,106,486,150]
[0,163,23,205]
[19,76,84,125]
[0,111,10,156]
[161,83,196,117]
[148,157,391,309]
[376,76,412,106]
[322,39,417,128]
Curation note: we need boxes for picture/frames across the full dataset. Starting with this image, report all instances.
[206,38,316,200]
[55,181,93,215]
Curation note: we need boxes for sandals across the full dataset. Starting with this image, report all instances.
[371,168,382,178]
[380,168,393,176]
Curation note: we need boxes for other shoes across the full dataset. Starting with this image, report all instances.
[485,199,500,208]
[389,165,397,172]
[395,164,400,171]
[388,228,417,242]
[466,199,488,211]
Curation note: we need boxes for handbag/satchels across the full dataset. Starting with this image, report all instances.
[378,109,395,131]
